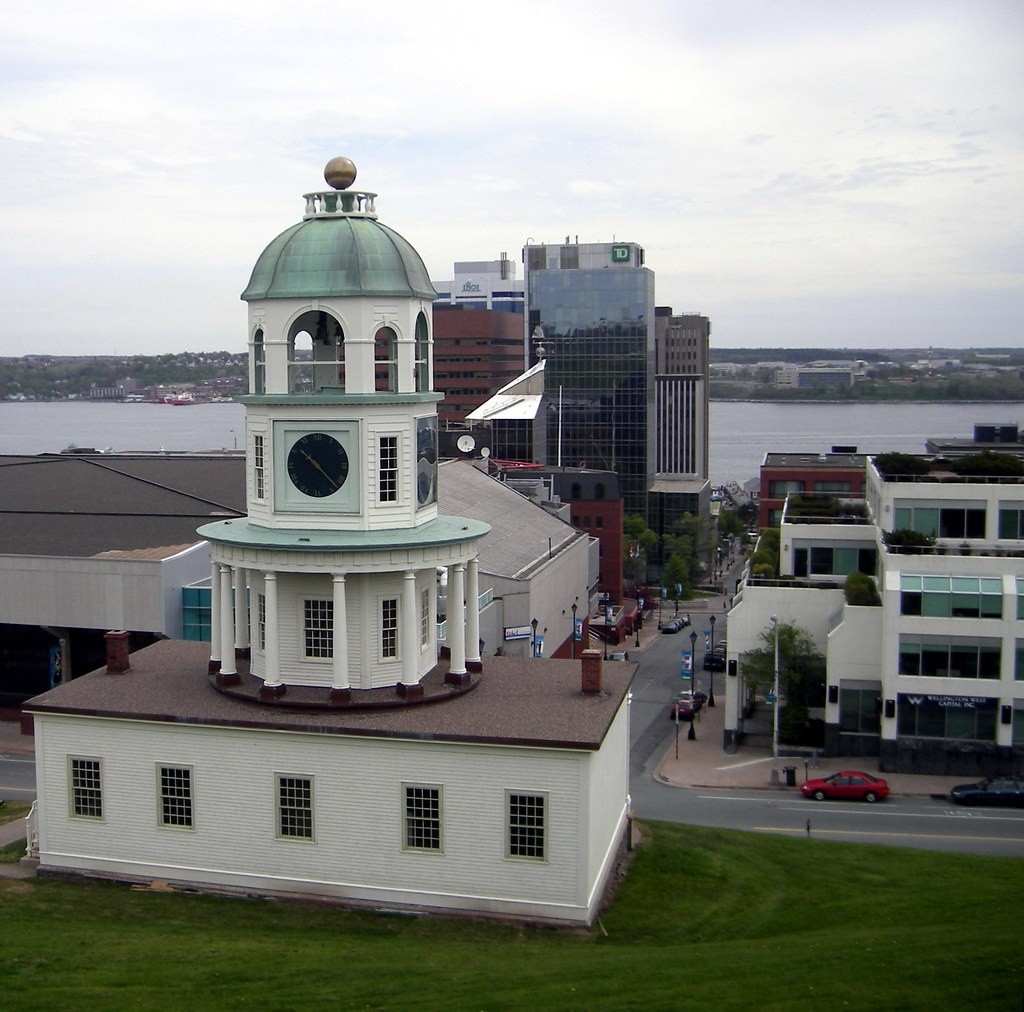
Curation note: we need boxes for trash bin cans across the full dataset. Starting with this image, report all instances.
[783,766,798,787]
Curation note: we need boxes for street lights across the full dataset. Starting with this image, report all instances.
[770,614,783,767]
[687,631,698,740]
[531,618,539,658]
[571,603,578,659]
[603,592,608,660]
[707,614,716,707]
[635,587,640,648]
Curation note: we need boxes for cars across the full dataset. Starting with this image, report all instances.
[800,770,891,803]
[950,776,1024,806]
[660,614,692,633]
[703,639,727,671]
[670,690,707,721]
